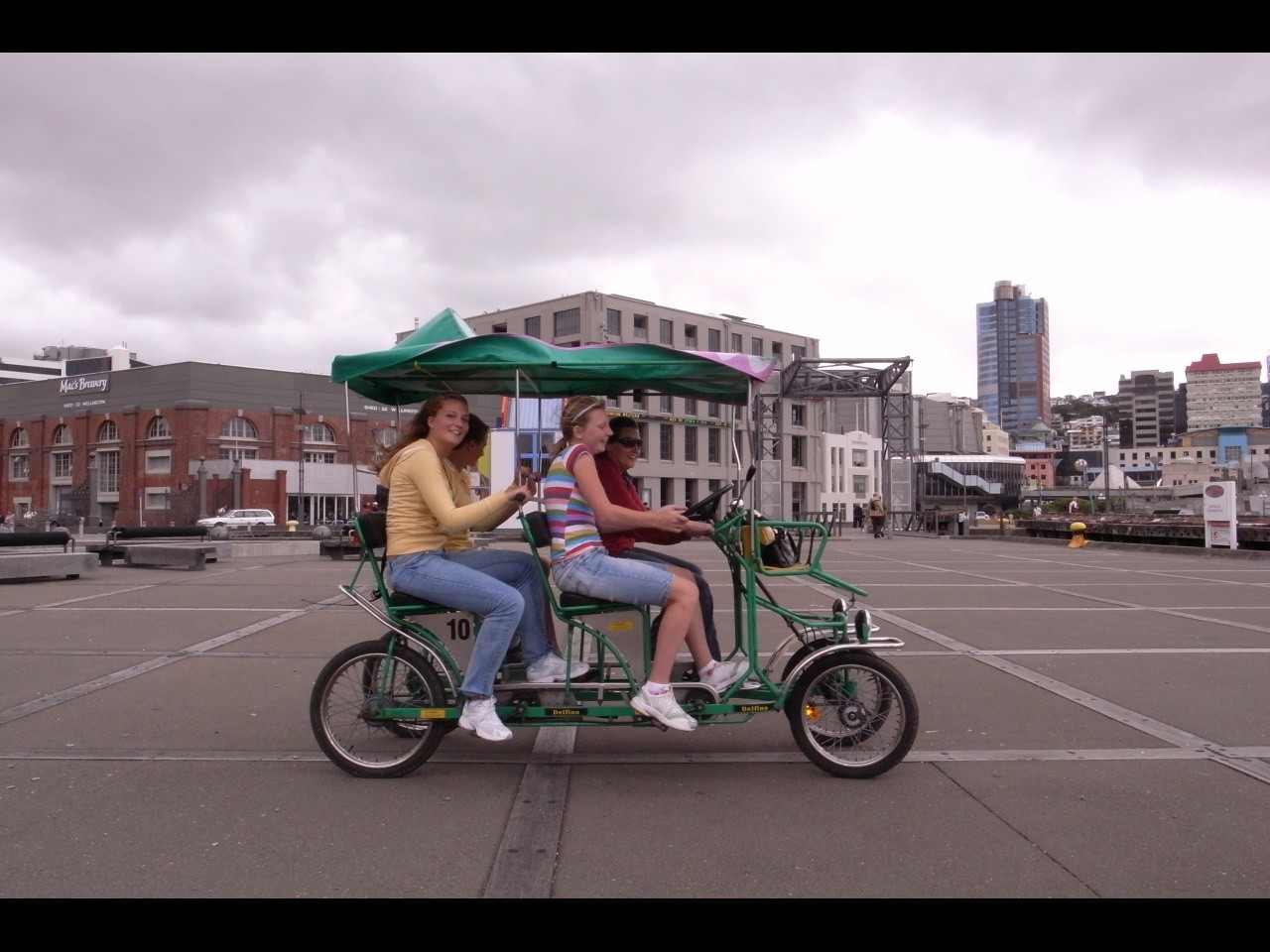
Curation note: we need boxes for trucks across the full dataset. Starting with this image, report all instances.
[958,511,990,522]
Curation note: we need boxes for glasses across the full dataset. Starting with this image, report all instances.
[611,438,643,449]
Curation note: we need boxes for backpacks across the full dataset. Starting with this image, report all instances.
[869,500,877,511]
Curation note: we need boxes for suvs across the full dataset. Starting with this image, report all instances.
[194,508,277,537]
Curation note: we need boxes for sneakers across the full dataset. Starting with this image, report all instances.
[700,660,749,693]
[526,652,592,684]
[630,686,697,731]
[458,696,512,741]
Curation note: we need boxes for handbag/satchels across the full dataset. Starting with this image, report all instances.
[761,528,797,568]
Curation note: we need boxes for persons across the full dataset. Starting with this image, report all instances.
[359,500,378,514]
[218,504,227,515]
[853,502,864,528]
[869,493,887,540]
[544,397,750,732]
[957,509,967,535]
[1068,498,1080,516]
[379,392,591,743]
[0,509,15,526]
[1033,505,1042,517]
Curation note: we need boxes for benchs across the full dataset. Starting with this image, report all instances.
[0,525,233,581]
[319,499,648,617]
